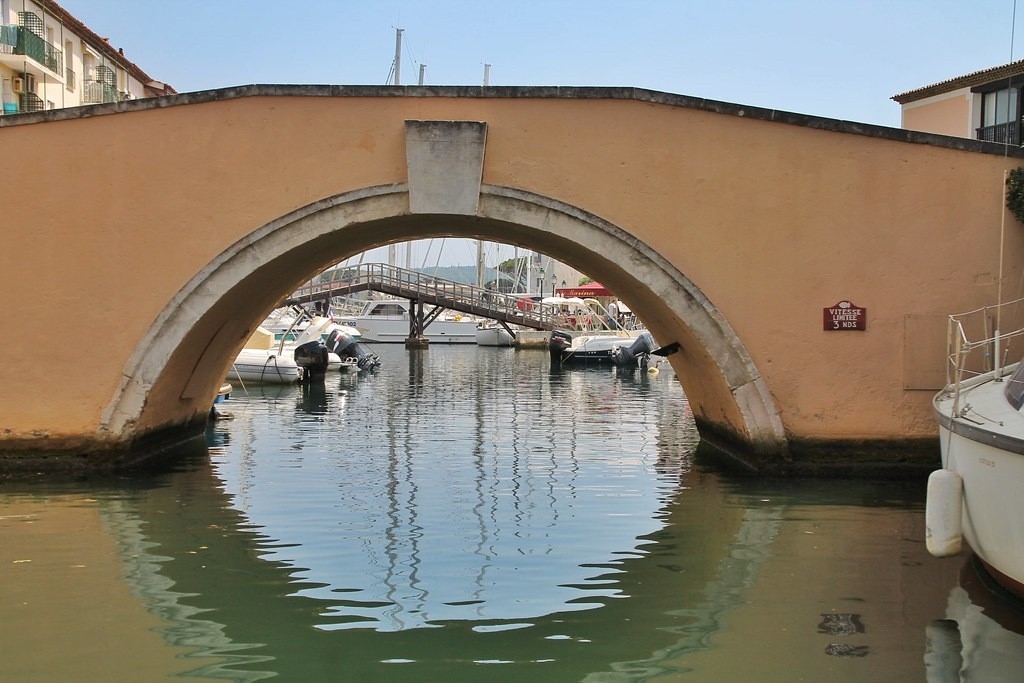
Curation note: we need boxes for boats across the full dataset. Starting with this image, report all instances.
[538,298,638,363]
[214,311,362,403]
[923,300,1024,591]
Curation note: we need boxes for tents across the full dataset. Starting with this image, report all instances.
[556,282,616,297]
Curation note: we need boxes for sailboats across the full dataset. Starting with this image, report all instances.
[475,248,575,347]
[260,28,498,343]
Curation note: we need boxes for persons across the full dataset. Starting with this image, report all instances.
[608,299,616,329]
[561,309,570,316]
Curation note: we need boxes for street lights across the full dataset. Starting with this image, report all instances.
[539,268,568,326]
